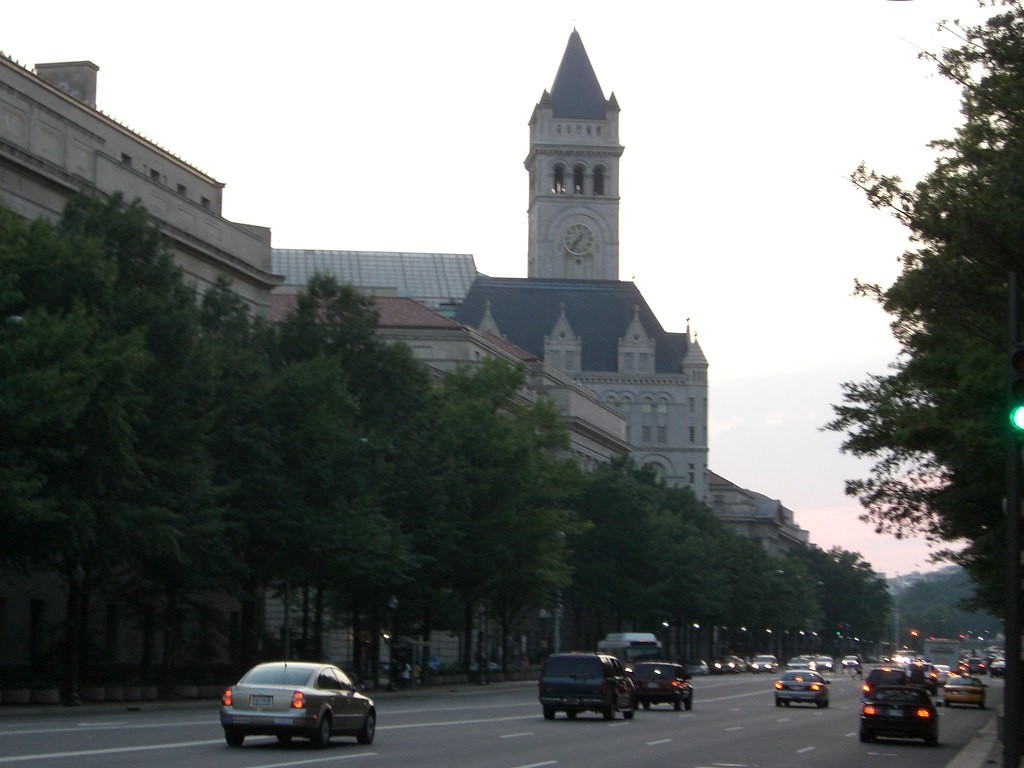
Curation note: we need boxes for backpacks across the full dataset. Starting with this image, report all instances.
[396,662,405,671]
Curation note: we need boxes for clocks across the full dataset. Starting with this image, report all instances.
[562,223,596,256]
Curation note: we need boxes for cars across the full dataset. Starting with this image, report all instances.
[774,668,831,708]
[690,644,1006,690]
[220,660,375,752]
[942,674,990,709]
[630,660,694,712]
[858,685,944,746]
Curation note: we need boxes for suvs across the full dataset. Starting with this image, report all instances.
[540,651,636,721]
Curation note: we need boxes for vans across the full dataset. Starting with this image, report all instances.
[599,633,667,666]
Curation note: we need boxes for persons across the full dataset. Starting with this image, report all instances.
[398,658,414,692]
[851,654,865,681]
[413,660,422,687]
